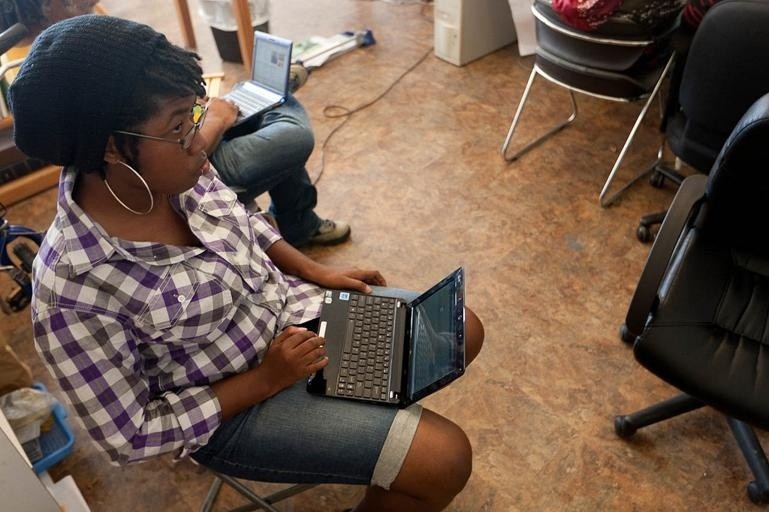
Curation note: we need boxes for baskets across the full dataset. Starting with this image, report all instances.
[29,384,73,474]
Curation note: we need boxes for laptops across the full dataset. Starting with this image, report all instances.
[219,30,293,127]
[306,266,466,409]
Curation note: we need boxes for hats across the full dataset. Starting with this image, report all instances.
[9,14,165,171]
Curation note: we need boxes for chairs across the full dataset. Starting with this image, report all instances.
[636,0,769,246]
[500,0,689,208]
[614,92,769,505]
[199,469,322,512]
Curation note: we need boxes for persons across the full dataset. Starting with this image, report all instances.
[2,0,351,249]
[9,12,485,511]
[554,0,712,133]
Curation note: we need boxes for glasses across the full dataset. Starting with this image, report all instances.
[113,101,208,150]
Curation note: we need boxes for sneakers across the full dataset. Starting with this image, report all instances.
[293,218,350,246]
[288,64,308,94]
